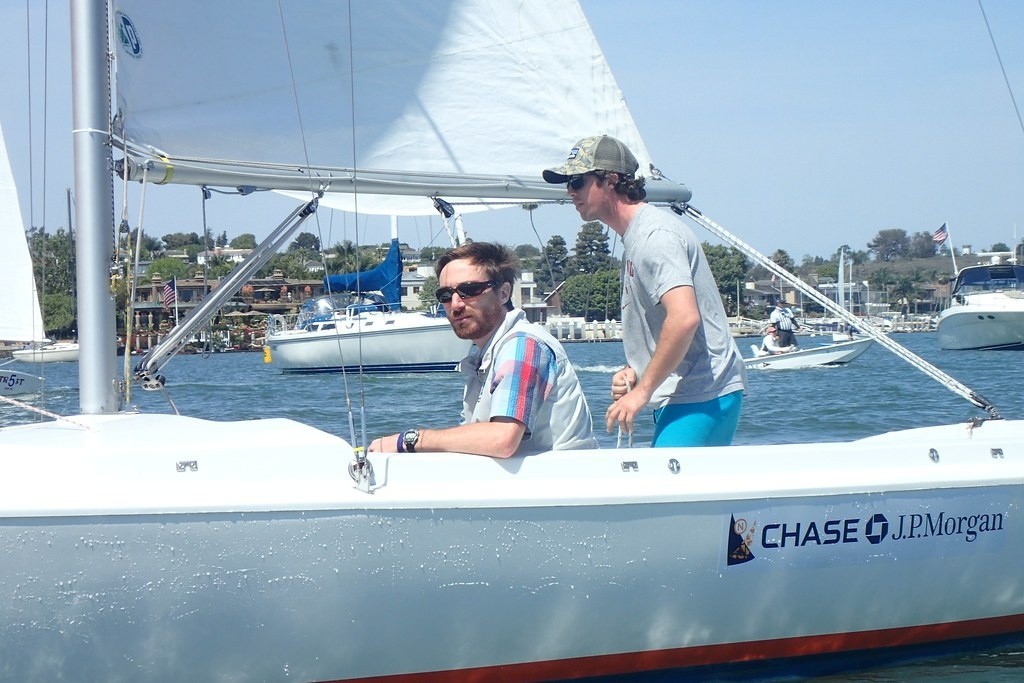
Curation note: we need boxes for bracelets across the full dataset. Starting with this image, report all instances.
[397,432,404,453]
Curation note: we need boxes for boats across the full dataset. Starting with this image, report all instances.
[740,336,874,369]
[934,223,1024,352]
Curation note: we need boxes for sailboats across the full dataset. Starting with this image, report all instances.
[0,128,51,395]
[261,187,513,374]
[10,189,81,362]
[0,0,1024,683]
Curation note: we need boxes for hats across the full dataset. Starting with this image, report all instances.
[776,297,787,302]
[542,134,639,183]
[766,326,777,333]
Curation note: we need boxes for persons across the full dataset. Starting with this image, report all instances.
[542,136,747,446]
[370,242,601,459]
[761,327,796,354]
[770,299,800,347]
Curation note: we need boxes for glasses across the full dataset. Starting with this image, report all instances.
[435,279,496,303]
[567,173,606,189]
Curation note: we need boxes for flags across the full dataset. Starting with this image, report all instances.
[164,279,175,307]
[932,224,948,244]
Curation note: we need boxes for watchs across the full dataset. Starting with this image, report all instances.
[404,429,420,453]
